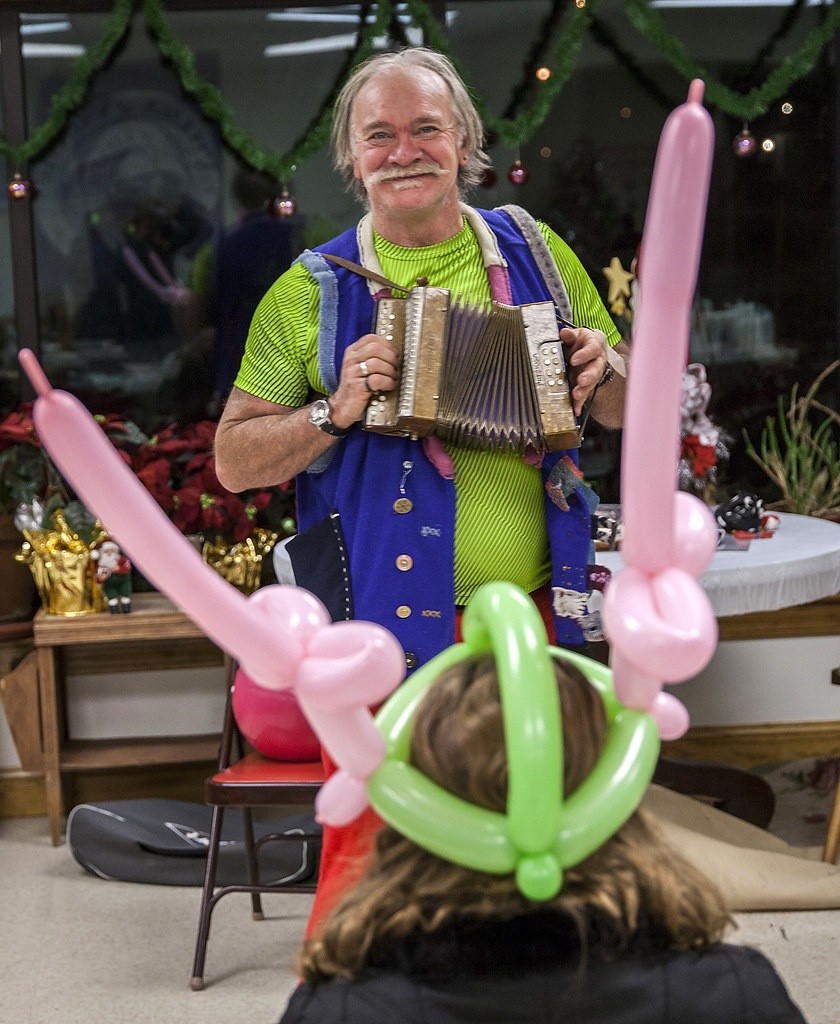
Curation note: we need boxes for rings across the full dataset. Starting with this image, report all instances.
[360,361,369,378]
[365,376,375,393]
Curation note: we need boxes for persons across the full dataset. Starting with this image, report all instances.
[276,651,807,1024]
[214,46,632,953]
[88,158,303,392]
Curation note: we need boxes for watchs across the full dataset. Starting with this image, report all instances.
[309,397,350,440]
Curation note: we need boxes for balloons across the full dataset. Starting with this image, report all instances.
[603,76,717,744]
[18,349,401,826]
[364,579,663,903]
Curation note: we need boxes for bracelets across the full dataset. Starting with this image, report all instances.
[593,363,614,389]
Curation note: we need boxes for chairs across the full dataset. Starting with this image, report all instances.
[189,649,324,989]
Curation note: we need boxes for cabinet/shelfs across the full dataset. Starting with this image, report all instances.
[33,592,840,865]
